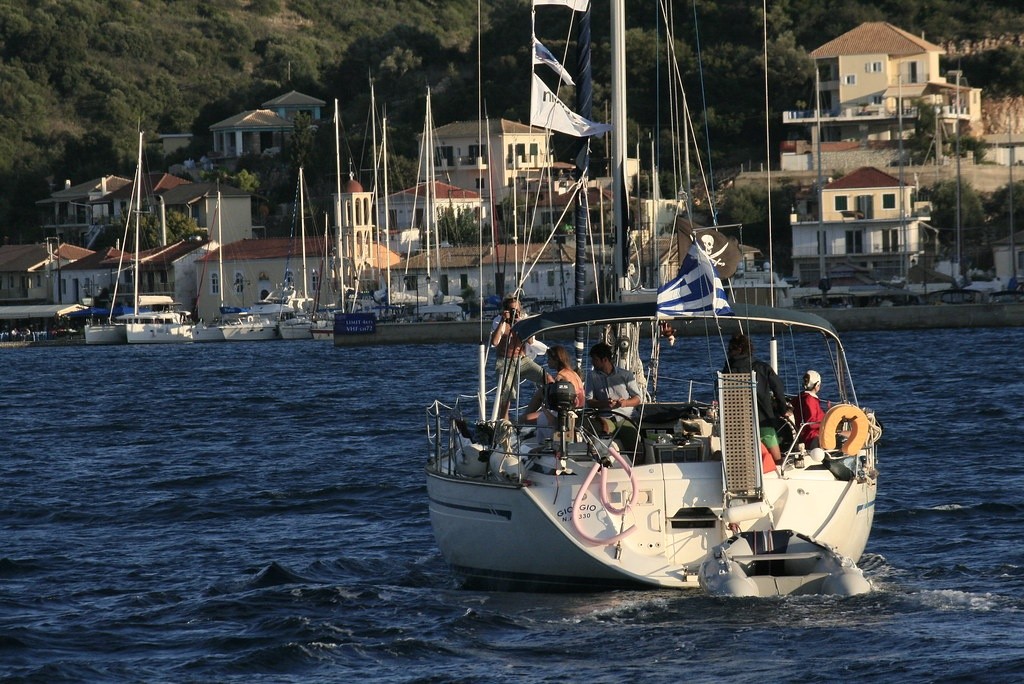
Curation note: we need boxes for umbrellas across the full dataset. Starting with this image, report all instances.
[68,307,150,327]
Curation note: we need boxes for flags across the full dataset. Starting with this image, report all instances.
[656,236,736,317]
[530,0,613,137]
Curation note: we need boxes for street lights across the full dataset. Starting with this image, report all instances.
[946,70,964,276]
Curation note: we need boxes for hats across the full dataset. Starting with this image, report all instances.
[802,370,819,391]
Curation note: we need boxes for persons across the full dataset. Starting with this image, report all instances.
[23,328,30,343]
[11,328,17,341]
[713,334,786,466]
[489,294,555,421]
[791,370,825,451]
[522,345,585,445]
[585,343,641,468]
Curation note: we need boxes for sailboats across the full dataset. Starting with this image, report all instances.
[424,0,879,603]
[82,0,1024,345]
